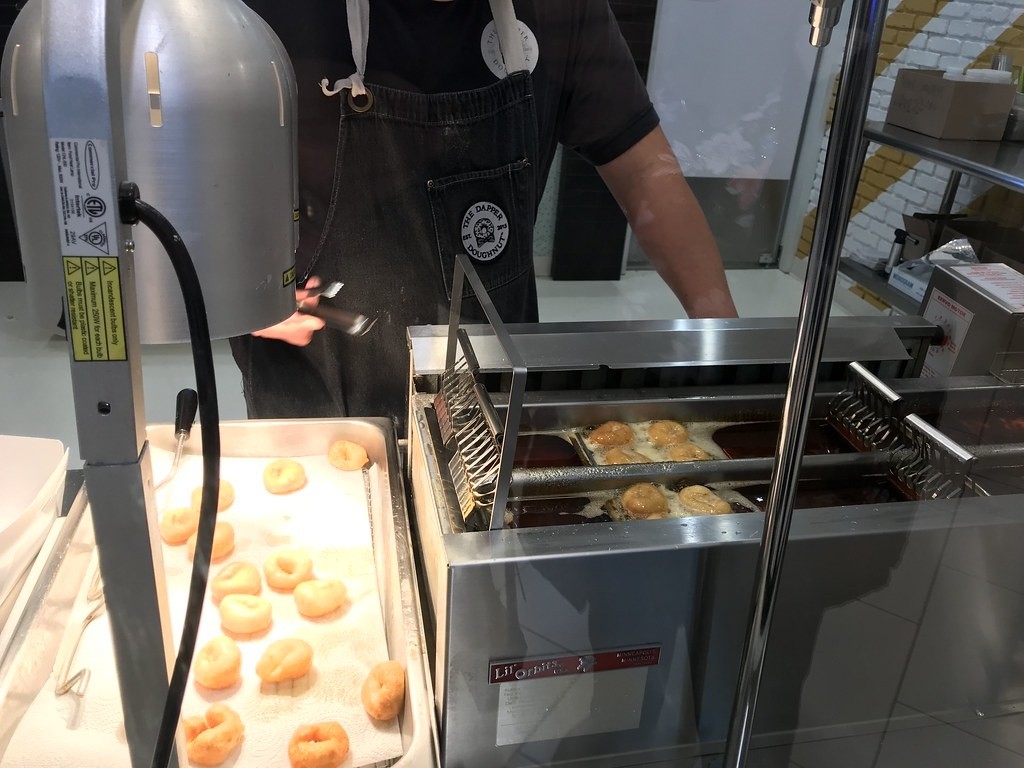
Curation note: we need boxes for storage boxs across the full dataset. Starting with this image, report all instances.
[935,219,1001,262]
[901,212,969,267]
[978,233,1023,274]
[885,69,1019,144]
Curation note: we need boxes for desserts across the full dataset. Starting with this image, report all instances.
[157,439,406,767]
[590,420,731,523]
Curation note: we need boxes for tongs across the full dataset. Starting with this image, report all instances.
[288,282,384,338]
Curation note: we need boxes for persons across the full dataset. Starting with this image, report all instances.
[208,1,743,438]
